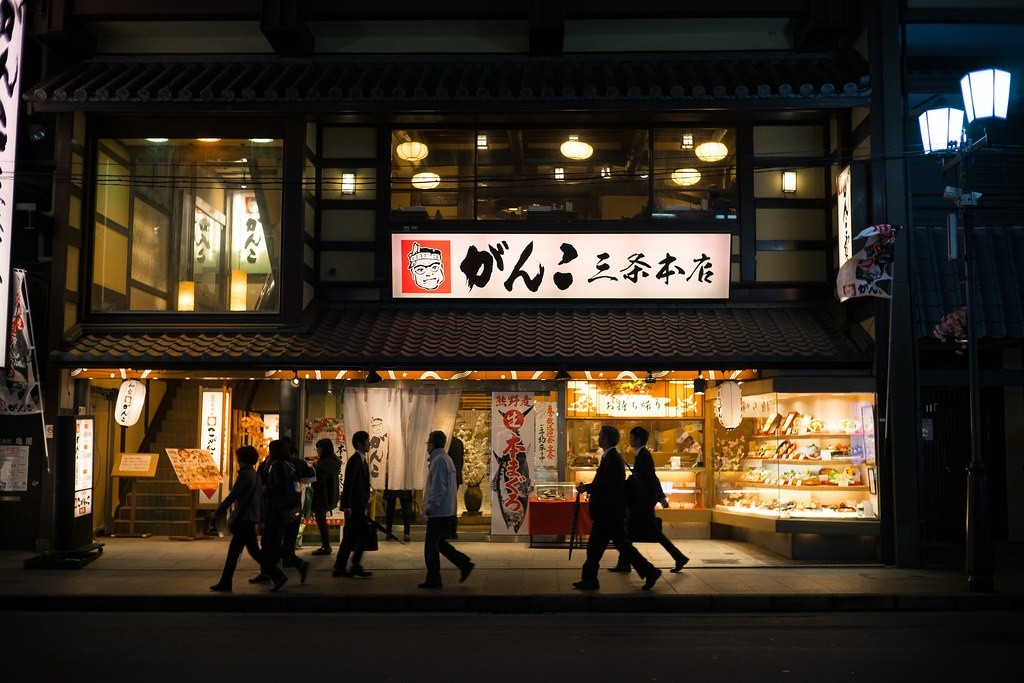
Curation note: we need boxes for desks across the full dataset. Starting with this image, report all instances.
[529,496,591,548]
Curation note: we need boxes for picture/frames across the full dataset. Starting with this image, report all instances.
[860,405,878,495]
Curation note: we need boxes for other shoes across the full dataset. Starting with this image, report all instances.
[333,569,351,577]
[404,534,410,542]
[299,562,310,582]
[642,570,662,590]
[248,575,271,584]
[386,532,392,539]
[460,562,475,582]
[210,581,232,592]
[417,583,442,589]
[573,580,600,589]
[670,557,689,572]
[350,569,373,579]
[314,546,332,555]
[607,564,631,572]
[270,574,288,592]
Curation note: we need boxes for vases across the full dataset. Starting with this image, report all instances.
[464,486,482,512]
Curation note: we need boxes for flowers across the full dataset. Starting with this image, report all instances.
[451,408,491,487]
[236,413,273,471]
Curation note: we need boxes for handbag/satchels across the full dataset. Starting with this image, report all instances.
[627,517,663,542]
[229,512,250,535]
[620,459,646,504]
[350,524,378,551]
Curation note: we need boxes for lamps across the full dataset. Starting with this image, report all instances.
[176,270,248,313]
[681,133,694,149]
[342,172,356,195]
[396,142,428,161]
[365,370,382,384]
[671,169,701,186]
[290,370,302,387]
[644,370,656,383]
[560,140,594,160]
[555,371,571,381]
[411,172,441,189]
[477,134,489,150]
[694,370,706,395]
[695,142,728,162]
[782,172,797,193]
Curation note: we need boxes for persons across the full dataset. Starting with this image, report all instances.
[311,438,341,554]
[333,431,380,581]
[571,425,661,592]
[208,444,289,592]
[383,447,413,541]
[681,432,702,453]
[606,427,690,571]
[417,429,476,588]
[445,432,464,492]
[247,436,310,583]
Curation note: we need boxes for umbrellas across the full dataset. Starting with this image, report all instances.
[568,482,584,559]
[361,512,405,544]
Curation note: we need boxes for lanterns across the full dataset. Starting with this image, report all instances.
[113,377,147,428]
[716,380,742,431]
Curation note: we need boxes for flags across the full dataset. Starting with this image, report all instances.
[0,267,42,415]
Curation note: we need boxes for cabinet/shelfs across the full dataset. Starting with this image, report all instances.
[568,466,712,539]
[711,434,878,560]
